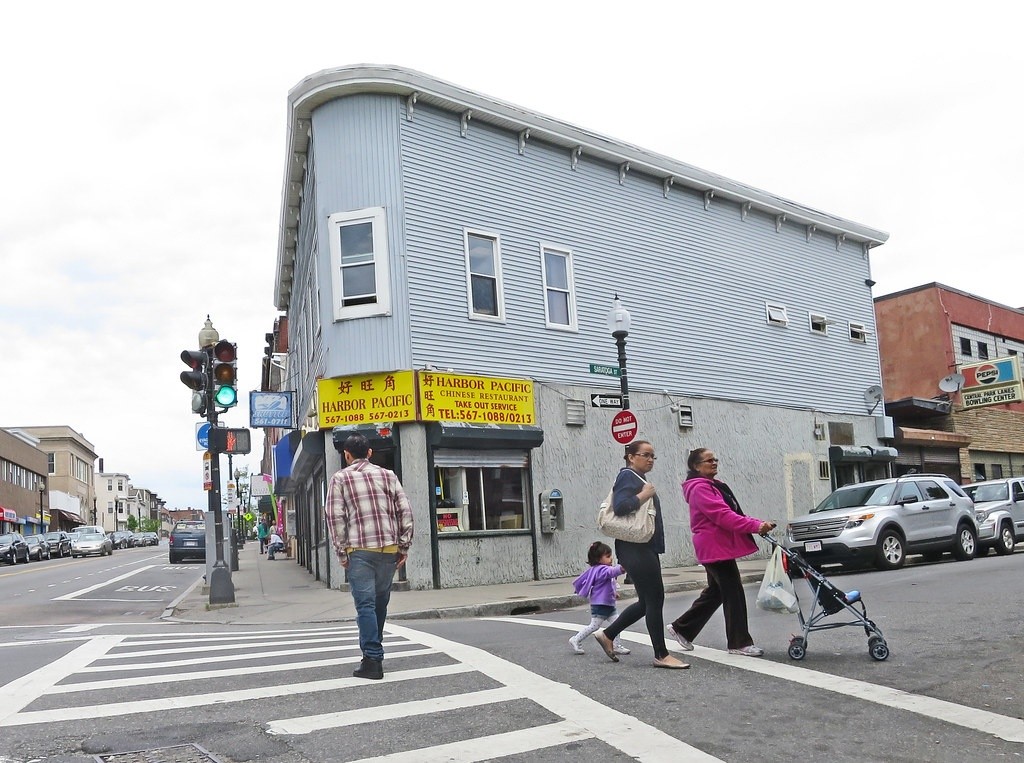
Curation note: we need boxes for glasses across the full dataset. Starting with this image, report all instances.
[700,458,719,463]
[632,453,657,460]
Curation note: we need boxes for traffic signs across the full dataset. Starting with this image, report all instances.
[588,363,621,378]
[588,393,626,409]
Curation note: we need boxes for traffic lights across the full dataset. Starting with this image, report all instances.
[213,338,238,408]
[179,347,210,415]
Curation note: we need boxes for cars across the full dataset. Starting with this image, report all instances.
[20,534,51,560]
[67,531,159,558]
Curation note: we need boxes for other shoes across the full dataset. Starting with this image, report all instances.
[259,551,263,554]
[594,630,619,662]
[653,658,690,669]
[265,551,267,554]
[268,556,274,560]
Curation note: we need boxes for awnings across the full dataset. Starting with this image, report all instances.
[59,510,87,524]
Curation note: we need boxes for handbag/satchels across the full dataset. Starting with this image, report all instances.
[596,469,657,543]
[755,545,800,616]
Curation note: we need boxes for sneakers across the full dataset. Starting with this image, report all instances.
[614,645,631,655]
[569,635,584,654]
[729,645,764,656]
[666,623,694,651]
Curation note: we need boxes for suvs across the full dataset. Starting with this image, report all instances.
[0,532,30,565]
[785,472,981,577]
[960,476,1024,557]
[168,519,206,565]
[42,531,72,558]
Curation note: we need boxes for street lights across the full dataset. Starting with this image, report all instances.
[114,496,120,532]
[92,495,97,526]
[234,465,247,549]
[609,294,637,468]
[137,503,142,528]
[38,481,45,535]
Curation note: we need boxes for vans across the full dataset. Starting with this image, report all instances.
[71,526,105,536]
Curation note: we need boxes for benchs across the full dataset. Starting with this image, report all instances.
[273,547,286,559]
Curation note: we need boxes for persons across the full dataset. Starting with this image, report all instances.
[568,541,632,654]
[258,519,269,554]
[324,433,414,679]
[665,448,773,656]
[264,534,284,560]
[595,440,691,669]
[270,520,277,534]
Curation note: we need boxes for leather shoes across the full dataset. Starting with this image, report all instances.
[352,660,383,679]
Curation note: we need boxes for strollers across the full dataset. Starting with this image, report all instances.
[759,523,890,665]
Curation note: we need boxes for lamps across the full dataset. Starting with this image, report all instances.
[939,373,966,393]
[865,385,883,414]
